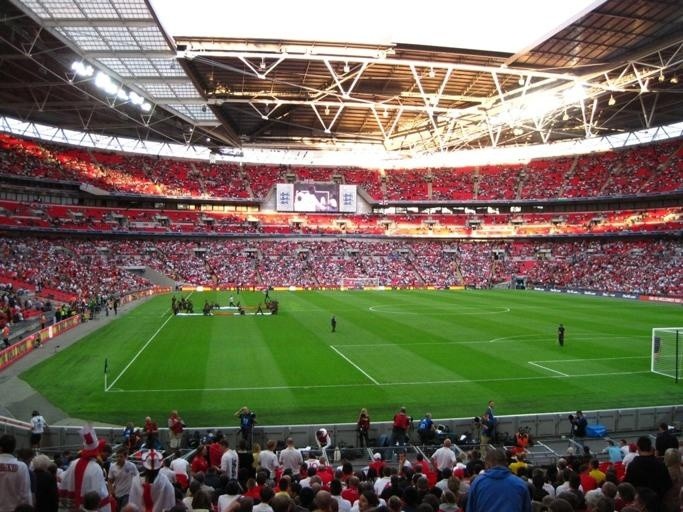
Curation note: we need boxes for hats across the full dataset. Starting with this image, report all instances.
[79,428,105,456]
[373,452,381,459]
[141,450,164,471]
[453,467,464,478]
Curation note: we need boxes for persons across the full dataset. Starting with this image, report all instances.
[1,131,682,346]
[1,400,681,512]
